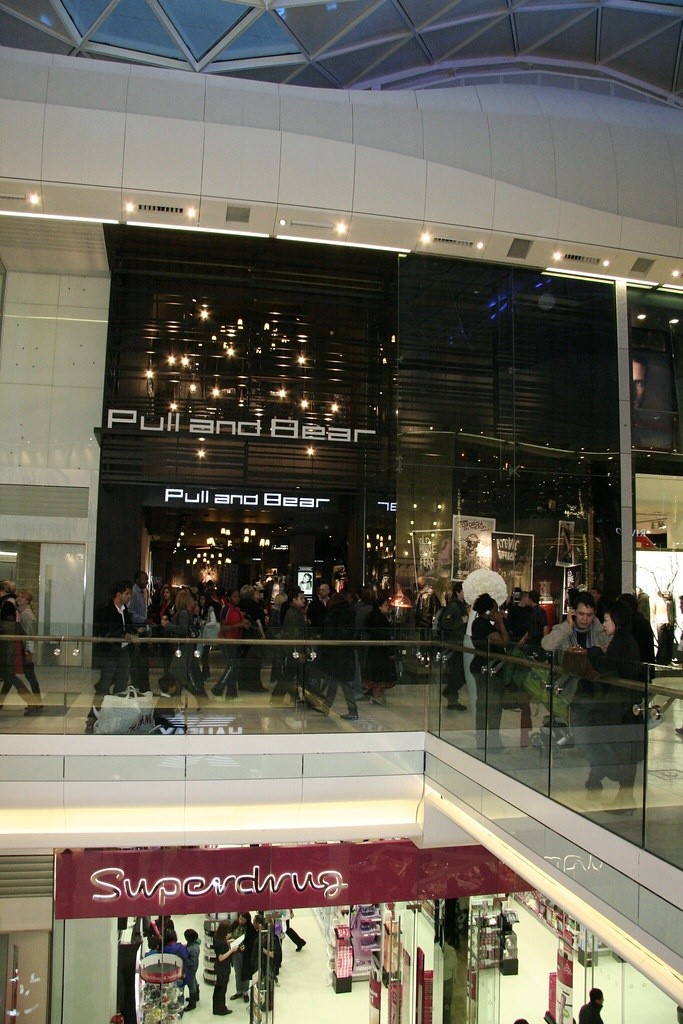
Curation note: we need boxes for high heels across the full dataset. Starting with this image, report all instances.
[602,790,637,816]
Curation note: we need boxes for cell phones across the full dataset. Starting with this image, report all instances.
[572,615,577,622]
[486,610,494,620]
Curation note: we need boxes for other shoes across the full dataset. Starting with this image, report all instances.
[242,994,250,1003]
[447,701,467,712]
[294,699,307,707]
[294,939,307,952]
[211,683,222,696]
[184,1001,197,1011]
[23,709,42,717]
[247,686,268,693]
[312,705,329,716]
[186,997,199,1002]
[476,736,486,749]
[270,699,291,708]
[224,696,244,703]
[213,1009,233,1015]
[442,688,459,702]
[238,685,247,691]
[584,780,604,793]
[84,725,95,734]
[488,735,506,754]
[230,993,243,1000]
[519,731,532,749]
[340,710,359,720]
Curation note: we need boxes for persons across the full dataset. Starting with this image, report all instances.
[0,579,43,715]
[151,913,607,1023]
[82,571,683,817]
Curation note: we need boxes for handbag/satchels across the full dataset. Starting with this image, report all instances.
[432,605,445,632]
[92,686,155,735]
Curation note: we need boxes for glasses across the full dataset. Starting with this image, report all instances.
[318,588,330,592]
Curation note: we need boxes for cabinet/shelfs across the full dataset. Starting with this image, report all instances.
[203,920,217,984]
[312,903,384,982]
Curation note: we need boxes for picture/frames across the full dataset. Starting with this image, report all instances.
[451,516,497,582]
[488,531,534,593]
[411,529,452,598]
